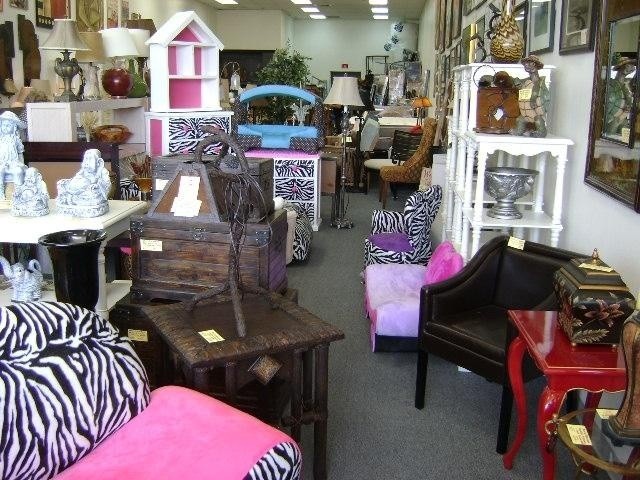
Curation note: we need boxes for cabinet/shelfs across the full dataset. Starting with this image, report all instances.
[151,112,232,158]
[147,11,224,114]
[26,99,149,185]
[233,150,323,232]
[440,61,576,268]
[0,196,148,325]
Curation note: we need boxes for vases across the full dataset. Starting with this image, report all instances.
[36,225,109,315]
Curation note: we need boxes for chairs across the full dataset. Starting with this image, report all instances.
[366,242,465,356]
[364,129,422,200]
[363,184,443,265]
[412,234,600,456]
[0,302,304,480]
[380,118,439,199]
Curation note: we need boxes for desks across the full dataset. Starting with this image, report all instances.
[140,289,345,480]
[541,409,640,479]
[501,307,631,480]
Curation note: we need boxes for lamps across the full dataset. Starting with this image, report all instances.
[322,73,366,230]
[218,58,245,92]
[128,29,151,99]
[98,27,139,102]
[75,30,107,101]
[38,19,92,105]
[411,95,432,127]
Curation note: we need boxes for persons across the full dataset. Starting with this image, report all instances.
[56,148,112,204]
[0,111,27,199]
[13,166,50,212]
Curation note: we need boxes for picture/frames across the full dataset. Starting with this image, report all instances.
[583,2,639,212]
[432,0,601,95]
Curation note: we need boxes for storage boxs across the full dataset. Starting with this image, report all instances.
[553,247,637,353]
[129,210,290,302]
[151,152,276,223]
